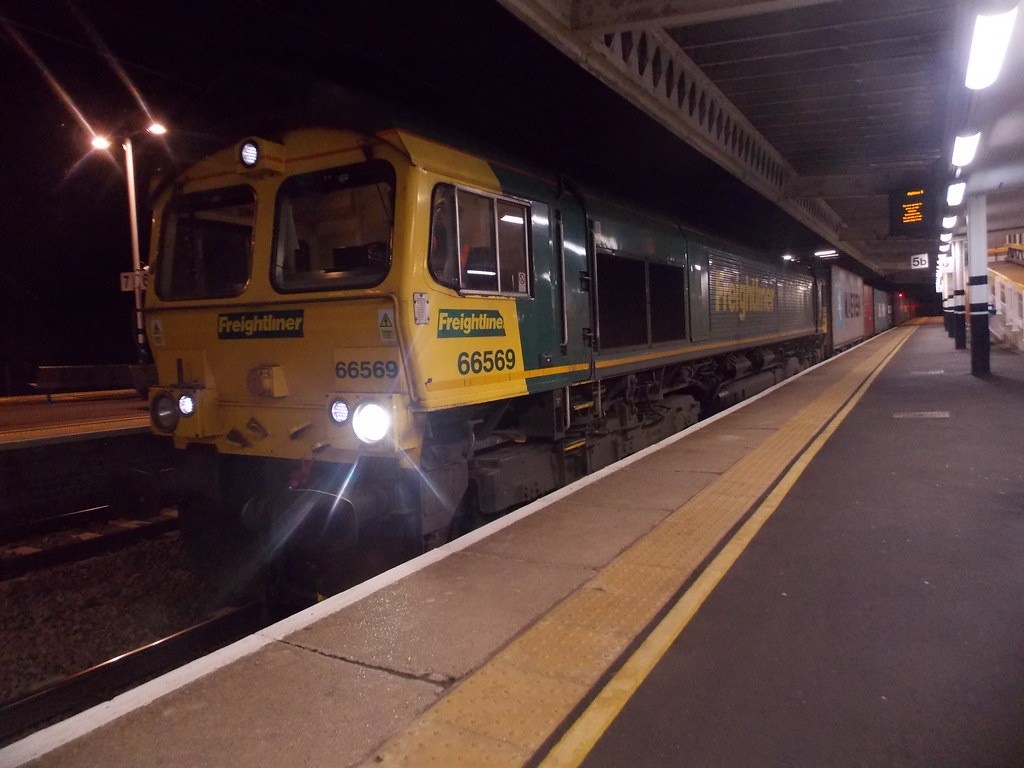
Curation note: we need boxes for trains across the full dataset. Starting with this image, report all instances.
[139,126,924,521]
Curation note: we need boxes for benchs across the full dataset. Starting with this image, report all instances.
[27,362,157,403]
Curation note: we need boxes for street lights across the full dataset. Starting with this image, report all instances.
[91,121,170,401]
